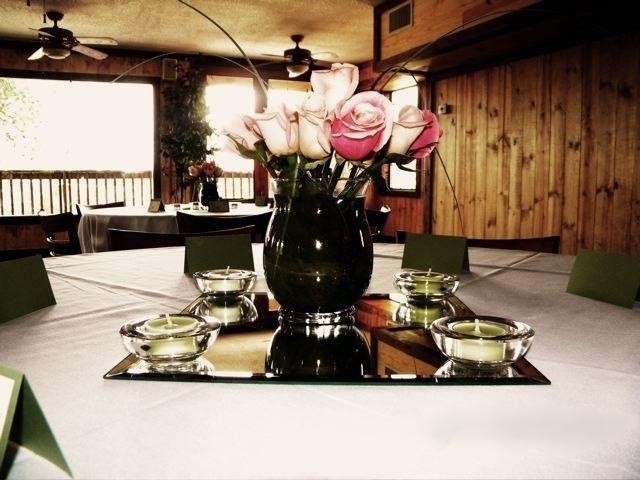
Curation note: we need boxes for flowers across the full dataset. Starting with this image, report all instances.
[110,0,542,278]
[188,161,222,184]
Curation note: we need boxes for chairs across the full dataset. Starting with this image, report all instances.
[38,209,81,256]
[106,225,256,251]
[76,202,124,217]
[365,205,391,242]
[394,230,560,254]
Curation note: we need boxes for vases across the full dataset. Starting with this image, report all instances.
[263,178,377,381]
[199,182,219,206]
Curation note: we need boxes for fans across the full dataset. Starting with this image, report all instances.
[27,11,118,60]
[244,36,330,74]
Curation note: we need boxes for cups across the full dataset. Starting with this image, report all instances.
[173,201,238,213]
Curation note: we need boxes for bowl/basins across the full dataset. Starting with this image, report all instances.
[142,356,215,375]
[192,267,256,296]
[393,302,455,329]
[430,313,534,371]
[431,362,523,380]
[117,314,222,360]
[199,294,261,328]
[393,271,462,302]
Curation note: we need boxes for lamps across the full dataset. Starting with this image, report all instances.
[41,48,70,59]
[287,64,309,74]
